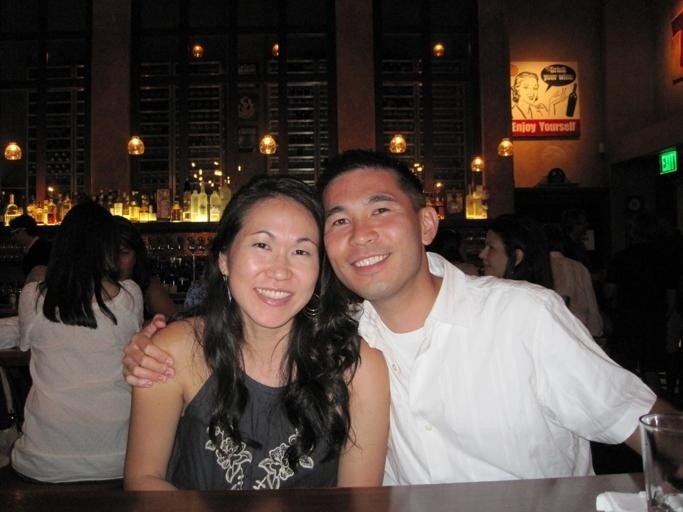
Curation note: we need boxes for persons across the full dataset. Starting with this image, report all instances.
[124,176,392,490]
[478,214,552,289]
[11,200,145,483]
[111,214,177,325]
[121,147,683,487]
[9,213,57,288]
[540,217,605,347]
[510,72,568,120]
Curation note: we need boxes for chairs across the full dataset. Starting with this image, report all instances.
[550,250,620,356]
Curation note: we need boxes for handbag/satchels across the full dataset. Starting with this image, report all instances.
[0,367,23,473]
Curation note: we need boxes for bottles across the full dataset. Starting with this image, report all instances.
[92,181,232,224]
[26,191,78,225]
[465,184,475,218]
[3,194,17,226]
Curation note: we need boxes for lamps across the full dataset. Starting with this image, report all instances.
[4,139,21,160]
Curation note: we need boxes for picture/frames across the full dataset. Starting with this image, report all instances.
[509,58,581,141]
[236,125,258,152]
[465,195,487,219]
[445,190,464,215]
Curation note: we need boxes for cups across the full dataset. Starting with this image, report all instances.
[640,413,683,512]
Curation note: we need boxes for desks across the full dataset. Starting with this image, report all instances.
[0,474,683,512]
[0,317,30,431]
